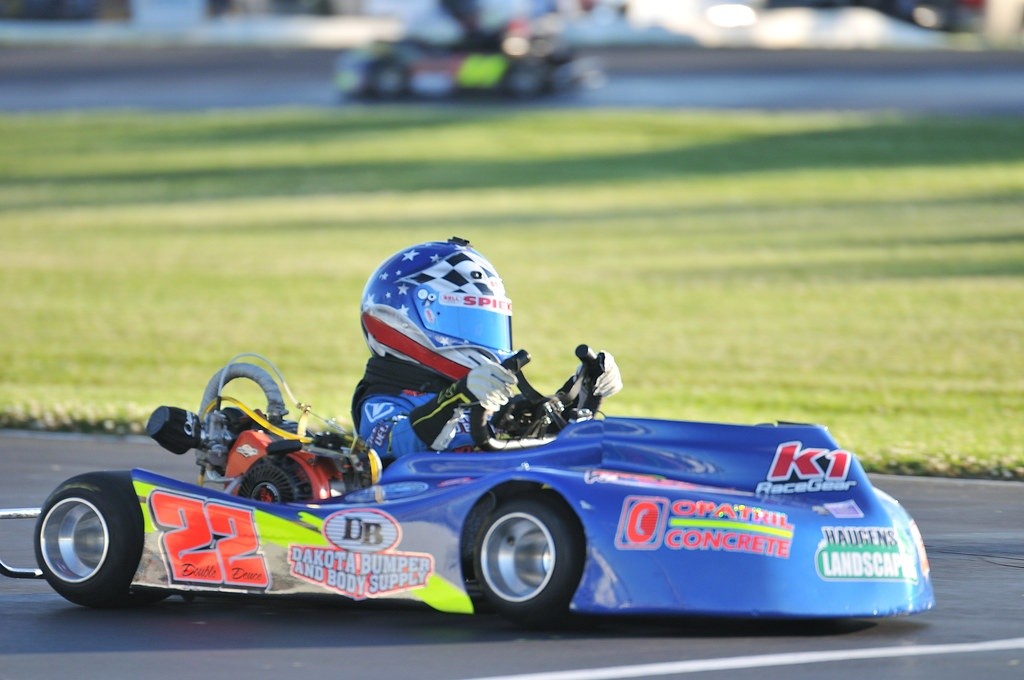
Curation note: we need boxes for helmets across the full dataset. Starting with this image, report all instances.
[360,235,512,382]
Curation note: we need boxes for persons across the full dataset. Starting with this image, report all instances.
[351,234,623,463]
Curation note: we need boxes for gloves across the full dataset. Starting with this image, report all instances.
[466,363,518,411]
[576,350,624,398]
